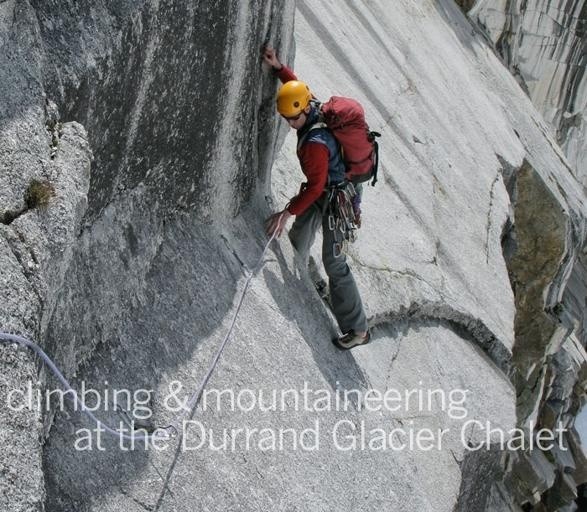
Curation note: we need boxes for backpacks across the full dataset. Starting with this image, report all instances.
[297,96,376,183]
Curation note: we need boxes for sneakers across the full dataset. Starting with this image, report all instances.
[333,328,370,350]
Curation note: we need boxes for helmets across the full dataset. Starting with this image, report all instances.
[277,80,313,115]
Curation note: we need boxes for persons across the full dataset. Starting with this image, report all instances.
[261,46,372,350]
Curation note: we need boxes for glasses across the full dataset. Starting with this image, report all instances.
[281,111,304,120]
